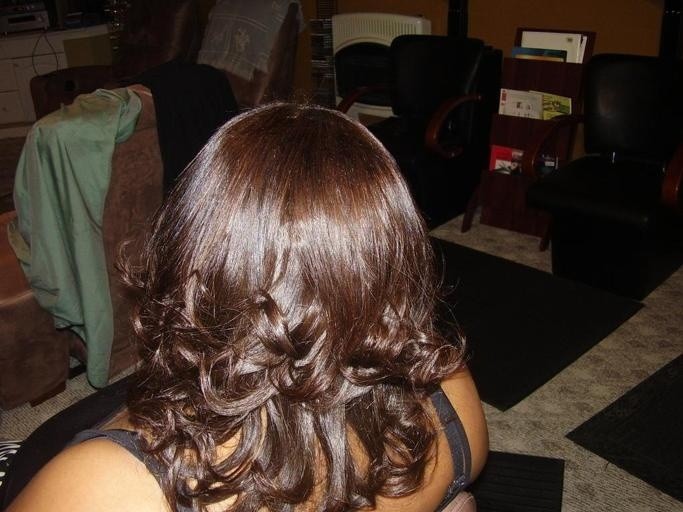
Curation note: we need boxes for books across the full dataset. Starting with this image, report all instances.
[487,142,559,183]
[521,31,582,63]
[579,35,589,64]
[509,47,567,62]
[527,87,571,125]
[499,87,544,121]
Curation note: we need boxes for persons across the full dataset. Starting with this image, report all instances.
[0,102,491,511]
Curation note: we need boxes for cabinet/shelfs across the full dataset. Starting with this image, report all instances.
[0,23,68,141]
